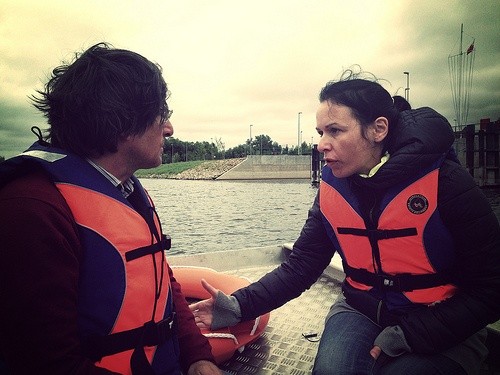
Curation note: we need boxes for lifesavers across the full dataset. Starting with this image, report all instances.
[169,266,270,358]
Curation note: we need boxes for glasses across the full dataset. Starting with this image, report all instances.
[159,107,173,123]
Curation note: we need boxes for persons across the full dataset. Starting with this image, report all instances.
[0,42,225,375]
[187,64,500,375]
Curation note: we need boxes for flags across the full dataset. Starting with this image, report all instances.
[467,39,474,55]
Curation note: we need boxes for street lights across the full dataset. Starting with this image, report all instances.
[403,72,410,103]
[250,125,253,155]
[298,111,302,154]
